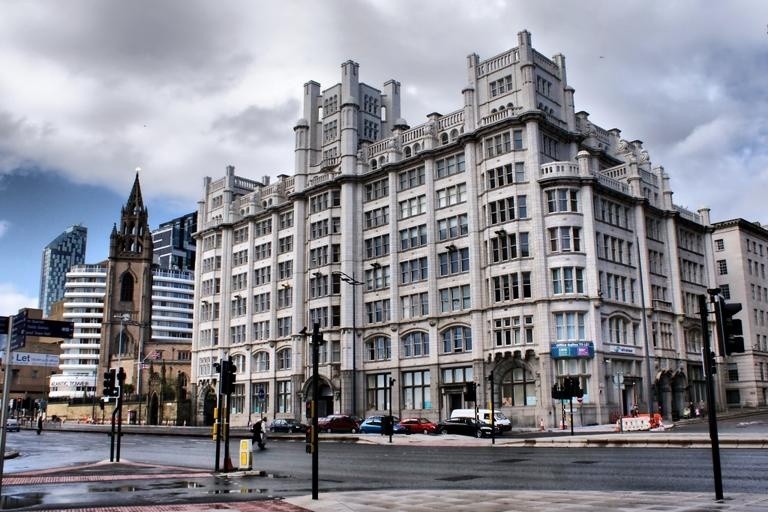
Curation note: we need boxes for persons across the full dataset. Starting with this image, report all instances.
[37,416,44,435]
[77,417,102,425]
[688,400,705,418]
[251,416,268,451]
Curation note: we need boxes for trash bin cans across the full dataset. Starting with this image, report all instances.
[127,410,137,424]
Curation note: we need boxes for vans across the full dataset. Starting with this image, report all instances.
[450,408,512,431]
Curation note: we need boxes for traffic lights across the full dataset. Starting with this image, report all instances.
[220,355,237,396]
[103,368,116,397]
[712,294,746,359]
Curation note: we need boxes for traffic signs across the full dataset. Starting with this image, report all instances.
[27,318,75,340]
[10,308,29,352]
[9,352,60,368]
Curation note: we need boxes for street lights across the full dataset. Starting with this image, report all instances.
[331,270,366,416]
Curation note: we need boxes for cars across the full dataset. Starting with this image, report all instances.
[437,417,500,438]
[359,416,409,435]
[397,416,438,434]
[6,419,20,433]
[270,417,306,434]
[317,415,362,434]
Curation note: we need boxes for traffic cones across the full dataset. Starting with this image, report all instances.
[614,419,621,432]
[538,418,546,431]
[558,418,564,430]
